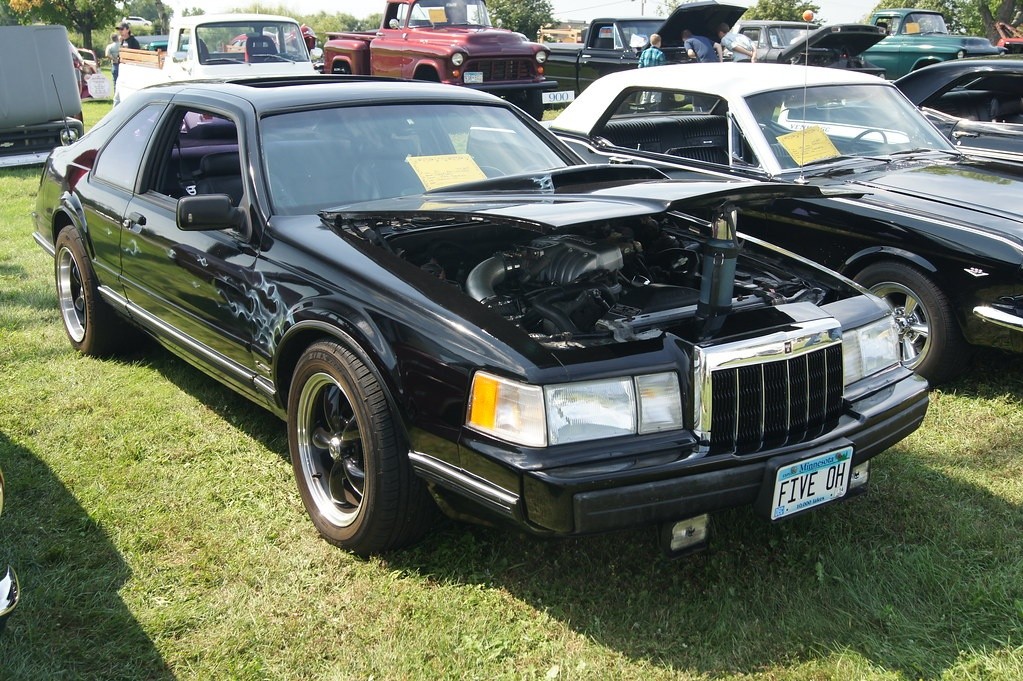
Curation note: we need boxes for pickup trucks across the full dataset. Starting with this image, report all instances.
[842,8,1007,92]
[323,1,550,126]
[533,0,747,115]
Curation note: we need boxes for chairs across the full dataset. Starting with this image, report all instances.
[350,132,426,204]
[195,150,245,206]
[244,36,278,63]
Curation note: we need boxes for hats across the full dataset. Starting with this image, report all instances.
[114,23,130,31]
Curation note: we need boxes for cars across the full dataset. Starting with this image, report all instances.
[72,47,100,74]
[33,73,937,555]
[543,59,1023,396]
[121,15,152,28]
[225,25,318,66]
[888,60,1023,178]
[995,35,1023,56]
[125,34,212,73]
[720,20,889,89]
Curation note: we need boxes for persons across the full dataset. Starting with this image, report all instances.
[115,22,139,49]
[637,33,665,104]
[105,34,121,93]
[681,30,724,112]
[717,23,758,62]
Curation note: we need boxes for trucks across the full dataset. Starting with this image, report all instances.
[107,12,315,120]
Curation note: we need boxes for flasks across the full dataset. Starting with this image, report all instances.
[696,208,739,316]
